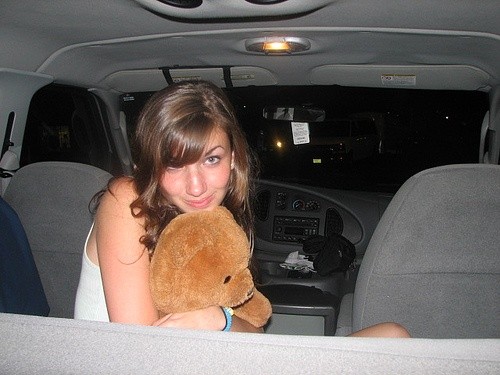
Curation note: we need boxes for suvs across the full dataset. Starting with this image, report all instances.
[261,111,380,173]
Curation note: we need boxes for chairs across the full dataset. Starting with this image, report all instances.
[334,163,499,338]
[4,161,114,319]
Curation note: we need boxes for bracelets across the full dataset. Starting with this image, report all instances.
[219,307,234,332]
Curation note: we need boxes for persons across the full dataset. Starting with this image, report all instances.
[74,82,413,339]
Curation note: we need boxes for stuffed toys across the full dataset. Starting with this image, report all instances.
[150,206,273,329]
